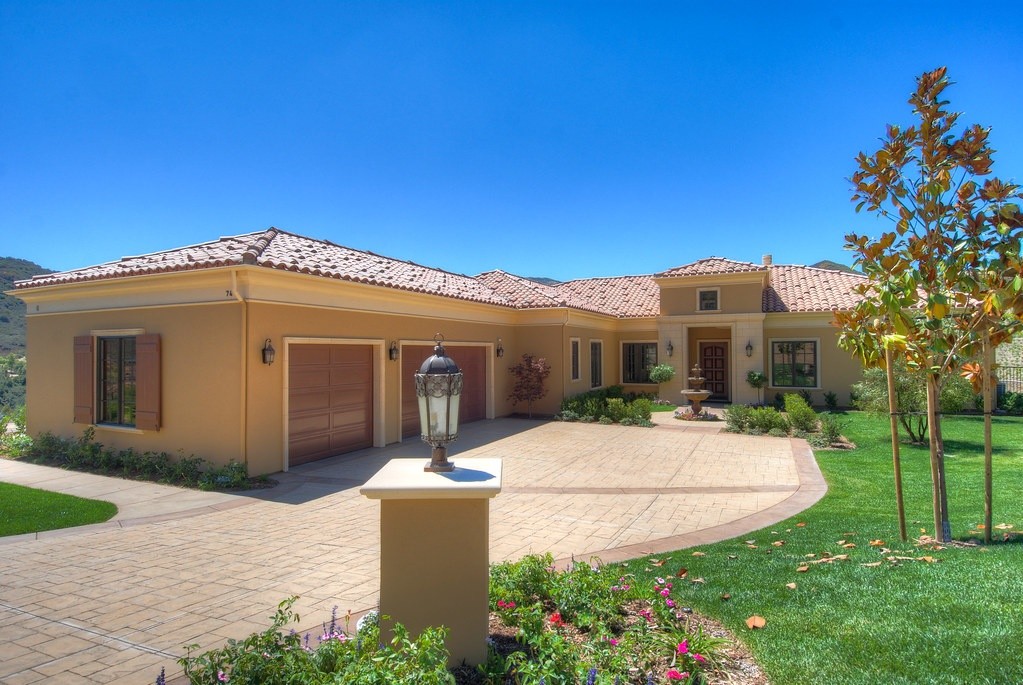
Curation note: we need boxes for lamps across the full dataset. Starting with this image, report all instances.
[262,338,275,366]
[666,341,674,356]
[496,342,504,360]
[746,341,753,357]
[410,332,463,472]
[389,341,401,361]
